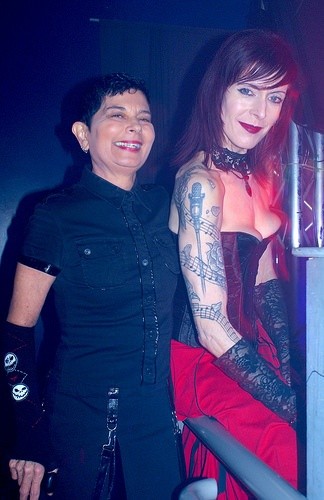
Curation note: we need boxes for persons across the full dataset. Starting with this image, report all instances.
[164,28,297,499]
[1,67,187,500]
